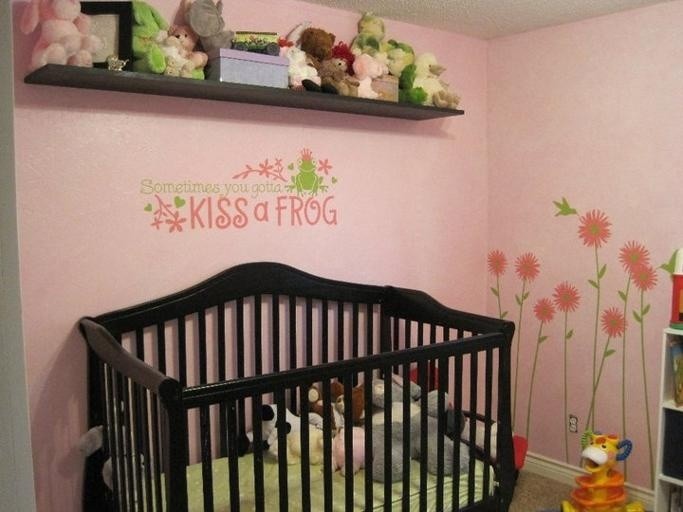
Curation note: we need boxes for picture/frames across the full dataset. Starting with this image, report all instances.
[78,0,136,73]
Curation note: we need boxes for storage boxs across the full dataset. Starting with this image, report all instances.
[371,73,400,103]
[204,48,292,89]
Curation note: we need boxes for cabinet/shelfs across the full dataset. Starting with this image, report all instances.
[652,328,683,510]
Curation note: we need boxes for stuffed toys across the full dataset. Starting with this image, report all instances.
[17,0,104,76]
[284,13,461,110]
[241,376,471,483]
[16,0,464,110]
[78,404,145,496]
[129,0,233,81]
[562,429,652,512]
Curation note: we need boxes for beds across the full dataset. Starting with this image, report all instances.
[72,256,525,510]
